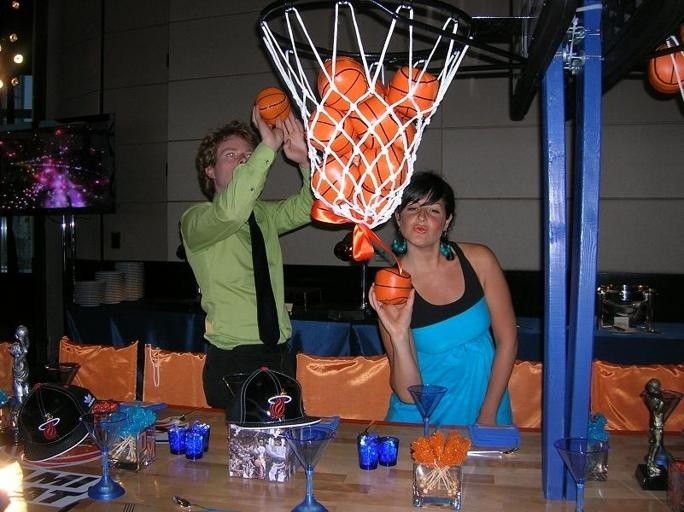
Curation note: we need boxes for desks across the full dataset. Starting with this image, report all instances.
[66,297,684,364]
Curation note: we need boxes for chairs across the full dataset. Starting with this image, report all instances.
[508,360,542,428]
[296,353,392,420]
[591,358,684,431]
[143,344,212,408]
[59,336,138,402]
[0,342,15,396]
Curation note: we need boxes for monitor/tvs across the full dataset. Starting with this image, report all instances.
[0,109,119,217]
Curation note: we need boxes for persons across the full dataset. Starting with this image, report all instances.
[368,171,519,427]
[178,104,314,411]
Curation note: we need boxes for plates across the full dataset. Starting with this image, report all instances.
[115,261,144,301]
[78,281,105,307]
[96,270,127,304]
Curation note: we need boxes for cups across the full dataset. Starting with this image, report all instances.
[379,437,399,466]
[184,432,204,459]
[193,423,210,450]
[407,384,447,437]
[80,411,128,499]
[358,432,380,469]
[286,426,333,512]
[45,363,81,386]
[641,390,684,465]
[554,437,610,512]
[169,426,187,454]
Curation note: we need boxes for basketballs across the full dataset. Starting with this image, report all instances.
[367,78,384,99]
[350,97,400,149]
[360,146,407,196]
[318,58,368,110]
[312,156,360,206]
[349,186,392,219]
[394,113,417,149]
[308,107,353,151]
[255,87,291,125]
[650,46,684,92]
[374,269,411,308]
[384,66,439,117]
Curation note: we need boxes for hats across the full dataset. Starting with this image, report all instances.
[227,367,323,429]
[17,381,99,463]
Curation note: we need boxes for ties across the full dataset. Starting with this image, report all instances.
[248,211,281,353]
